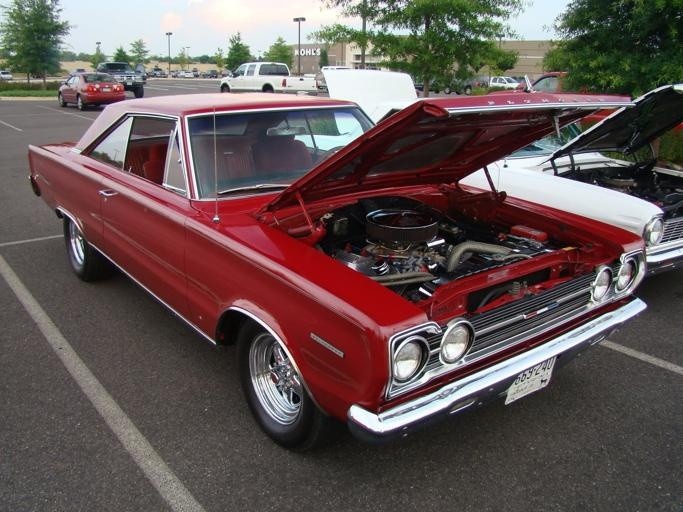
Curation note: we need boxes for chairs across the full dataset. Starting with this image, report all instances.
[125,135,312,185]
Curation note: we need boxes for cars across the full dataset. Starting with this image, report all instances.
[0,70,12,80]
[67,69,86,76]
[21,94,656,451]
[317,66,527,99]
[293,68,683,278]
[55,72,125,110]
[145,67,229,79]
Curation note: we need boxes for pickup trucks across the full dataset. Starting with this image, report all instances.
[219,61,316,96]
[486,71,682,133]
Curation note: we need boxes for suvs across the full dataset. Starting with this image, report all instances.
[97,61,146,98]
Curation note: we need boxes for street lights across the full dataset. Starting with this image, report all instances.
[185,46,190,69]
[165,32,172,75]
[95,42,101,48]
[292,17,305,74]
[495,33,506,51]
